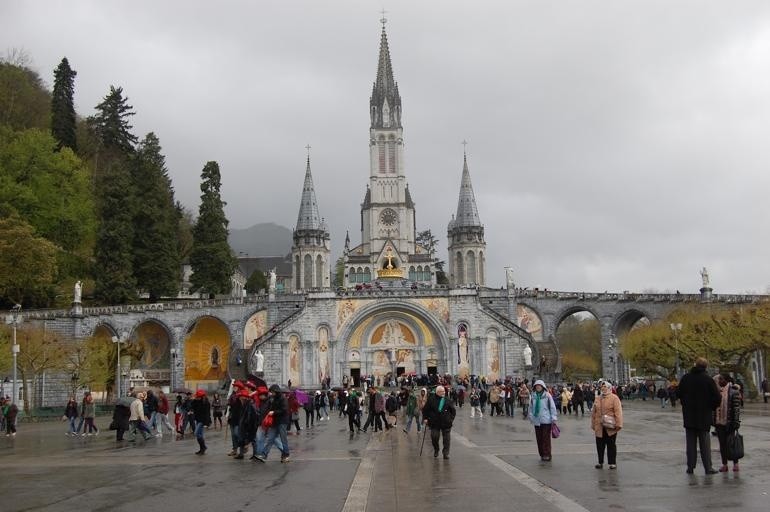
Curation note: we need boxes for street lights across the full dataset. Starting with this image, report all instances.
[6,303,23,408]
[110,332,132,400]
[171,347,180,393]
[670,321,684,383]
[608,333,620,387]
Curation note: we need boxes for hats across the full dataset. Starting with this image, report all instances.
[193,389,207,398]
[232,380,269,399]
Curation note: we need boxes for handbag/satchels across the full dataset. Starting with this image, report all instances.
[291,413,298,420]
[600,414,616,429]
[552,424,560,437]
[723,433,744,460]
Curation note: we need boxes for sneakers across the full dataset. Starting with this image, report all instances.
[64,429,99,436]
[541,456,552,461]
[317,416,330,421]
[733,463,740,471]
[116,438,136,442]
[443,454,450,459]
[195,446,207,455]
[350,424,398,432]
[705,468,718,474]
[417,431,421,433]
[470,415,482,418]
[686,468,694,473]
[249,454,266,463]
[145,429,174,440]
[281,452,289,463]
[490,412,513,417]
[403,428,408,434]
[5,432,16,437]
[434,449,439,457]
[306,424,313,427]
[719,465,728,472]
[228,445,249,459]
[595,463,603,469]
[609,464,617,469]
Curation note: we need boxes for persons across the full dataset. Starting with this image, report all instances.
[173,390,223,456]
[5,396,18,437]
[679,357,721,474]
[524,343,532,366]
[224,377,299,462]
[506,265,515,288]
[0,397,9,431]
[423,385,455,460]
[713,372,741,472]
[65,390,98,437]
[303,373,560,433]
[269,268,277,289]
[73,280,85,303]
[700,266,711,287]
[562,379,770,415]
[528,379,557,462]
[112,387,174,442]
[590,380,623,470]
[254,350,264,371]
